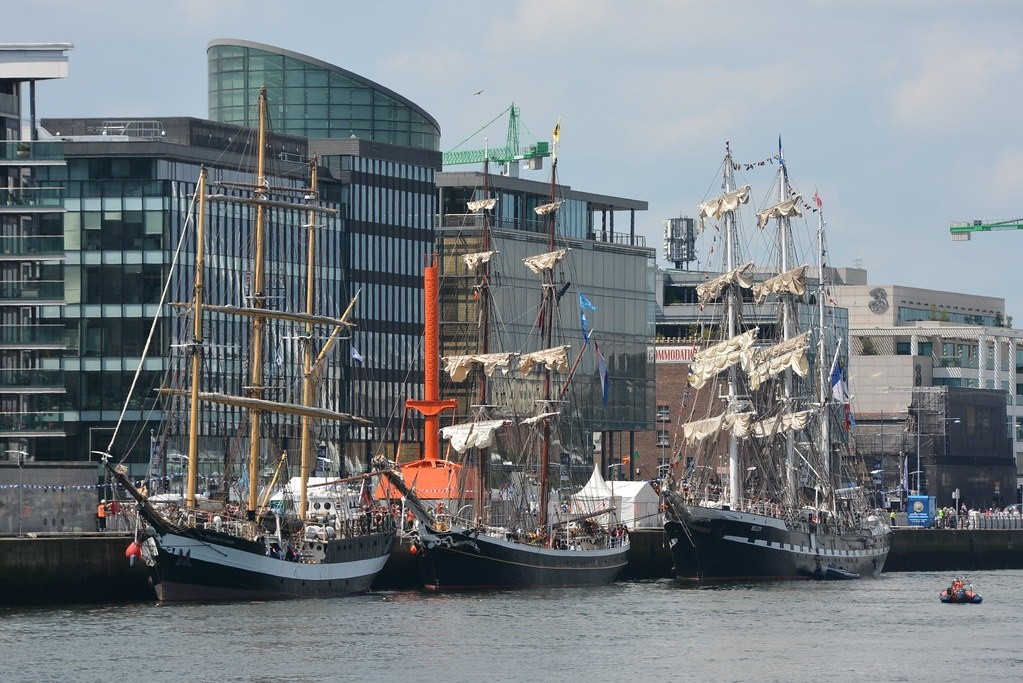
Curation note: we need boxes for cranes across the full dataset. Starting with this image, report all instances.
[442,103,553,178]
[949,218,1023,241]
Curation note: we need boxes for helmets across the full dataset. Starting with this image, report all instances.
[101,499,106,503]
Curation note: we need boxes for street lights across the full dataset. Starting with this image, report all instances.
[916,418,962,496]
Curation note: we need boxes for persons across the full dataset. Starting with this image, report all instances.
[97,499,113,531]
[889,503,1019,529]
[168,503,630,563]
[951,577,965,592]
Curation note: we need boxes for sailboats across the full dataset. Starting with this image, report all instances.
[90,80,400,604]
[337,158,630,595]
[651,132,894,585]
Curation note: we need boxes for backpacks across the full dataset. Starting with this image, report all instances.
[562,505,566,512]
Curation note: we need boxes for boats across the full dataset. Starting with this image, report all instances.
[938,586,982,603]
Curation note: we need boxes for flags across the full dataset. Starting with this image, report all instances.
[828,353,848,401]
[351,347,363,365]
[593,339,612,409]
[579,293,599,343]
